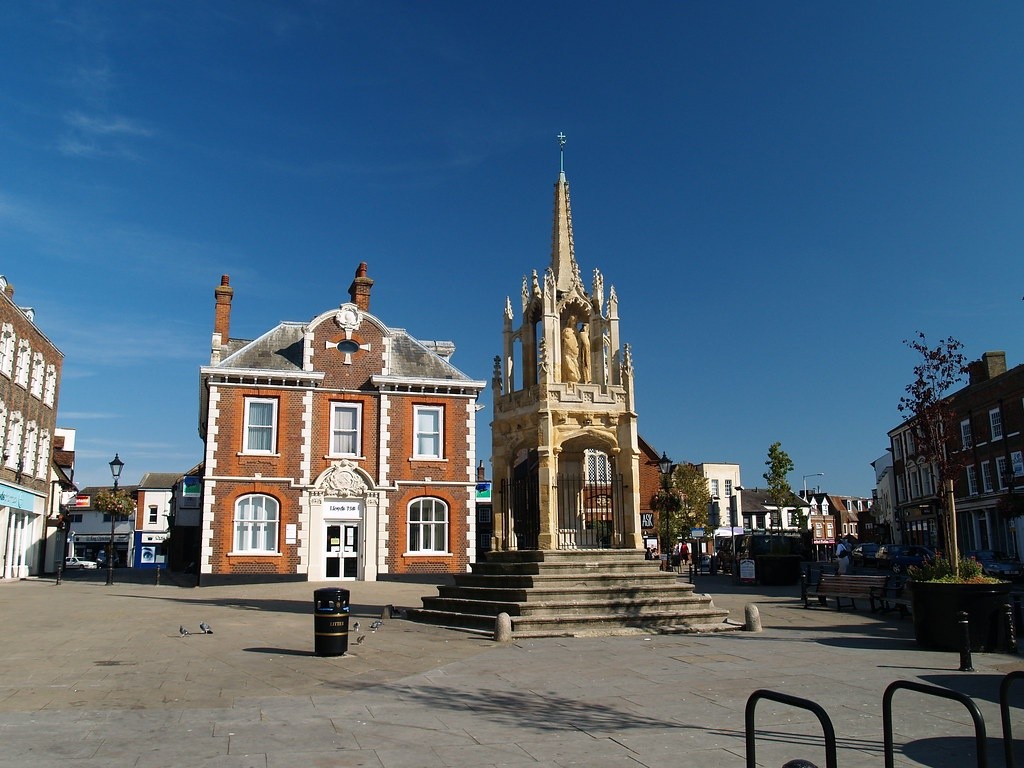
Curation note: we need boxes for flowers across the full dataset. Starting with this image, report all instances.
[649,487,684,515]
[92,488,138,515]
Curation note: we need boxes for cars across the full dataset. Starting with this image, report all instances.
[851,542,878,568]
[963,549,1024,585]
[876,541,939,574]
[66,556,98,570]
[701,555,712,567]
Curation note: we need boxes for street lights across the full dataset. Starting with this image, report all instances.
[709,493,720,576]
[105,452,125,587]
[802,471,827,503]
[657,451,673,573]
[728,485,744,557]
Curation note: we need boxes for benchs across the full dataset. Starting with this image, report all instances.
[803,574,893,617]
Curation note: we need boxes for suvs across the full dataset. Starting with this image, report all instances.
[94,548,121,568]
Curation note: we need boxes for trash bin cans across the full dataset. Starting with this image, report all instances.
[314,587,350,657]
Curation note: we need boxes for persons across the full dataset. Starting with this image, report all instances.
[645,547,659,560]
[834,537,852,573]
[680,541,689,565]
[561,314,591,384]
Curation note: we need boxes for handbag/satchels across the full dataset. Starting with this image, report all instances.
[837,544,848,558]
